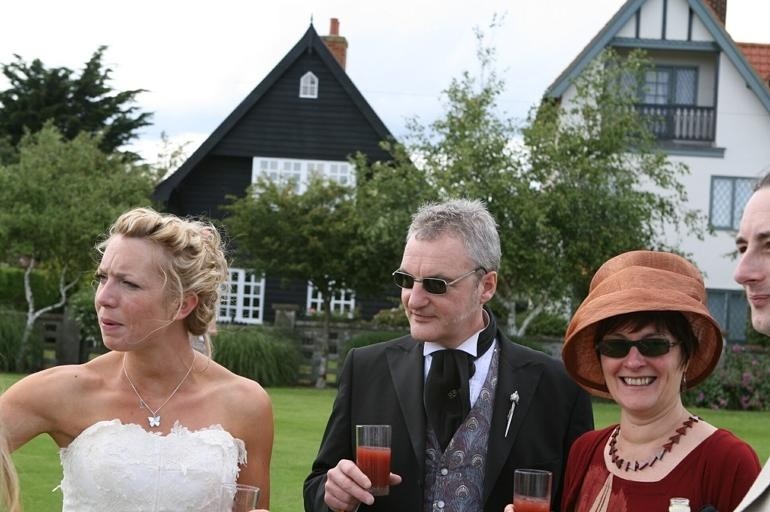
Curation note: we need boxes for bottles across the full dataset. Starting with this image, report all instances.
[669,498,690,511]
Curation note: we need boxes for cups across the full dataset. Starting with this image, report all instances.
[219,482,259,511]
[356,425,391,497]
[513,468,552,511]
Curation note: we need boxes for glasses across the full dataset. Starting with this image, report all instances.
[593,338,686,358]
[392,266,487,296]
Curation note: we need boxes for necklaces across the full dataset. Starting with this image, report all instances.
[123,350,197,427]
[609,412,702,472]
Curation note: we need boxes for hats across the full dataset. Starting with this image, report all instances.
[562,250,723,393]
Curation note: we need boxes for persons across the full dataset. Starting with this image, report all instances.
[0,205,275,510]
[0,431,20,510]
[495,251,761,511]
[732,170,770,512]
[303,199,597,512]
